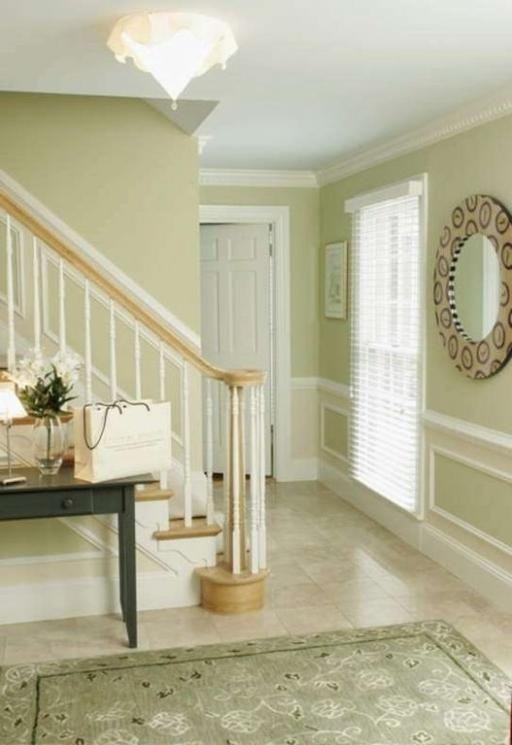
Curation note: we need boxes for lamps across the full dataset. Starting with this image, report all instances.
[107,8,238,110]
[0,389,29,484]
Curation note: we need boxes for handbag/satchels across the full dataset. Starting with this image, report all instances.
[72,398,172,483]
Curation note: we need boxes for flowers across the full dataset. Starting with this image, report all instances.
[10,344,85,469]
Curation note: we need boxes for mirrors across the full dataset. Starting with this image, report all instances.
[433,194,512,380]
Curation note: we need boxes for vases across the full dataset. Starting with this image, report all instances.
[31,415,65,476]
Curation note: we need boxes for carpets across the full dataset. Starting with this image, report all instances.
[0,619,512,745]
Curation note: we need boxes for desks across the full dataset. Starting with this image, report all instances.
[0,467,160,649]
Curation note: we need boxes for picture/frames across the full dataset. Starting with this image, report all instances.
[323,240,348,320]
